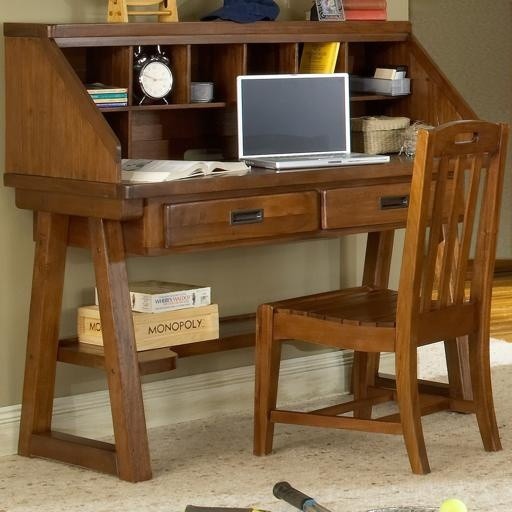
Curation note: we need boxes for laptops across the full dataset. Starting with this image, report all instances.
[235,73,392,170]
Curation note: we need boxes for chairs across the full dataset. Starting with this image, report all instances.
[252,120,505,474]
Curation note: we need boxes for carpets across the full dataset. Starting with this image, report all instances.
[0,349,512,512]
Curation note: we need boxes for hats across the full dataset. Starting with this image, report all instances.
[199,0,279,22]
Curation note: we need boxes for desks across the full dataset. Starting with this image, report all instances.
[4,15,489,484]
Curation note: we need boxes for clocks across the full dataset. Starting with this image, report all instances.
[134,46,177,105]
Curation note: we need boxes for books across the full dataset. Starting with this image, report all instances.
[93,98,128,104]
[311,0,388,10]
[121,157,252,183]
[95,103,128,108]
[298,40,340,74]
[83,82,128,95]
[305,10,388,21]
[90,93,128,100]
